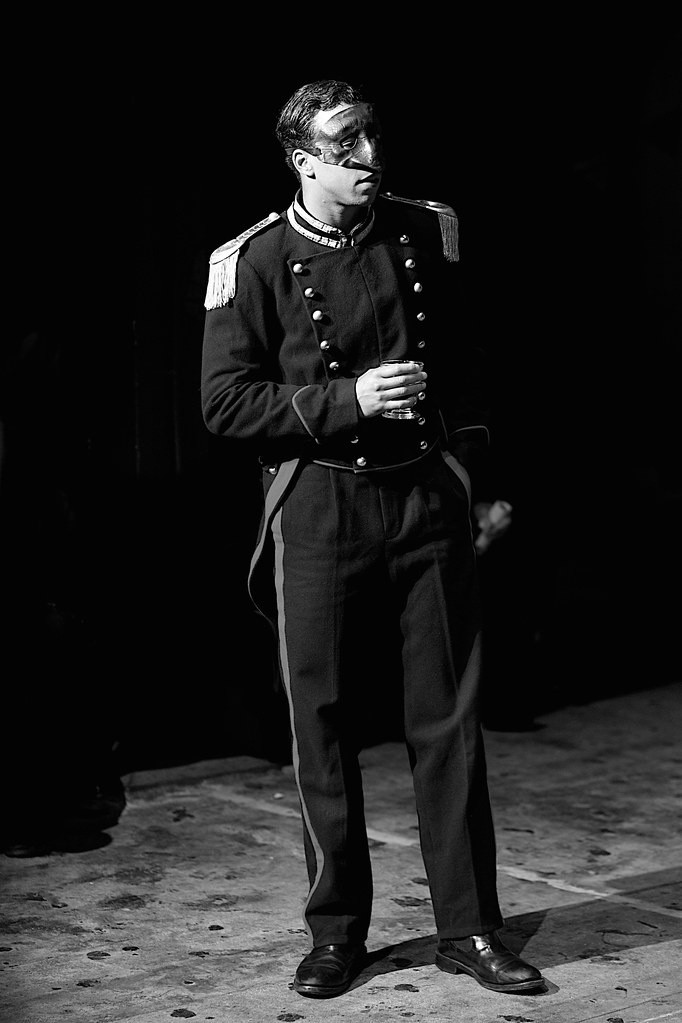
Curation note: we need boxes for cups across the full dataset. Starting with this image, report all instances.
[381,360,424,419]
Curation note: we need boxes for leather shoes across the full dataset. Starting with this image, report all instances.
[435,929,544,992]
[294,944,367,995]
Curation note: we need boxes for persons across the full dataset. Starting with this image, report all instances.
[201,80,544,999]
